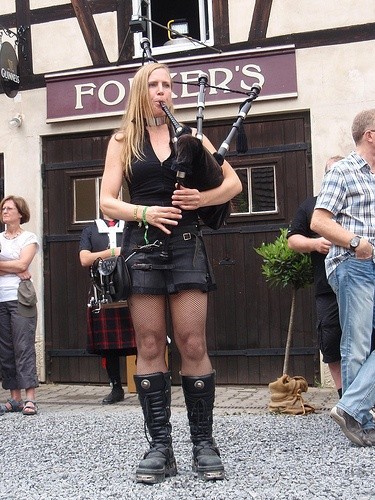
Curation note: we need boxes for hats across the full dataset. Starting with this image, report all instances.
[17,279,37,317]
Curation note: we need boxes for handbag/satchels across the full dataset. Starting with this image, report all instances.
[89,254,133,304]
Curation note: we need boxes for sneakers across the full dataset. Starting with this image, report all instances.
[329,406,375,447]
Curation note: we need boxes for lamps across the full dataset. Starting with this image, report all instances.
[8,113,22,131]
[167,18,190,40]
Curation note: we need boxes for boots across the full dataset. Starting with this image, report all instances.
[179,369,225,480]
[134,371,177,483]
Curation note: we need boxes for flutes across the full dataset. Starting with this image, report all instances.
[157,70,265,234]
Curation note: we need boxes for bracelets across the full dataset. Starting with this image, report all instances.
[134,205,141,227]
[142,206,149,244]
[111,248,115,257]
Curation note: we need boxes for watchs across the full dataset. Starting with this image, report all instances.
[350,236,362,252]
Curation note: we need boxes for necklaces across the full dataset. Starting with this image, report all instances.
[4,228,24,240]
[146,116,166,126]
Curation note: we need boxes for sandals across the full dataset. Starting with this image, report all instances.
[23,400,37,415]
[0,399,23,416]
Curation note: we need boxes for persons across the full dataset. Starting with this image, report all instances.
[79,211,138,405]
[310,108,375,448]
[285,156,375,399]
[101,61,243,483]
[0,195,40,414]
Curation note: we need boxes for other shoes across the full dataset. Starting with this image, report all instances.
[103,387,124,403]
